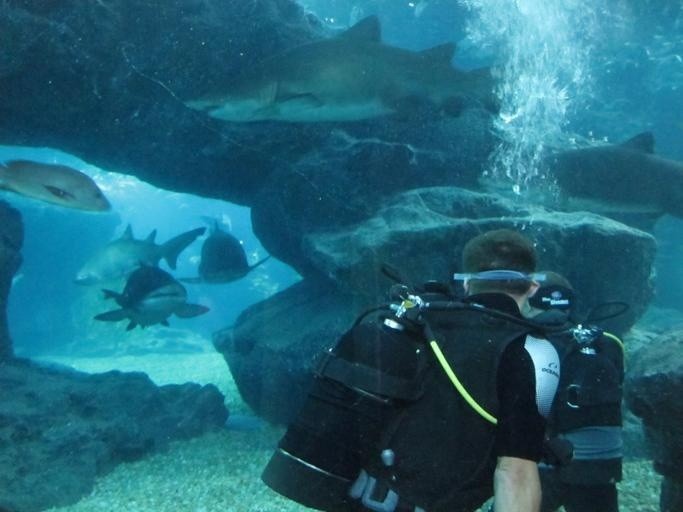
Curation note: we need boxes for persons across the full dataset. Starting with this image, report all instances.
[325,229,624,512]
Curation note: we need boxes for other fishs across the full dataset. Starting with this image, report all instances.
[1,157,111,212]
